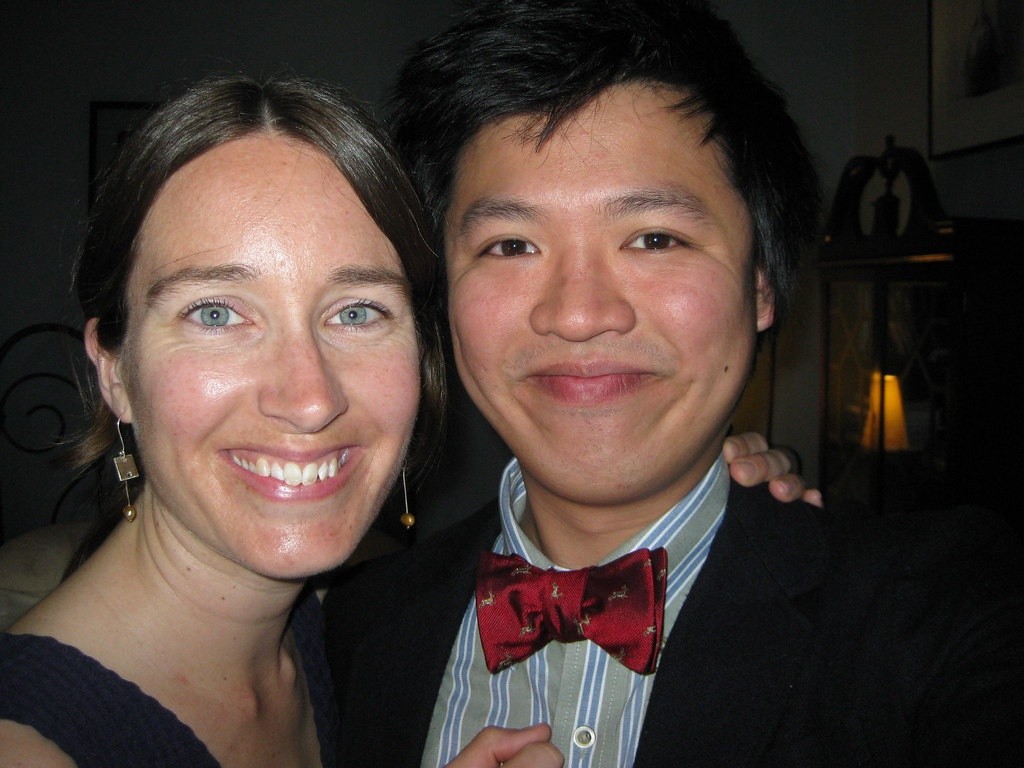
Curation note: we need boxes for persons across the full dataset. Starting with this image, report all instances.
[2,55,824,768]
[296,2,1024,768]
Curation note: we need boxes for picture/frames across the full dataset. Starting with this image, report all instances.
[89,97,170,208]
[926,0,1024,160]
[727,323,778,448]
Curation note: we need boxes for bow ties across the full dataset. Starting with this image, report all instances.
[474,546,668,676]
